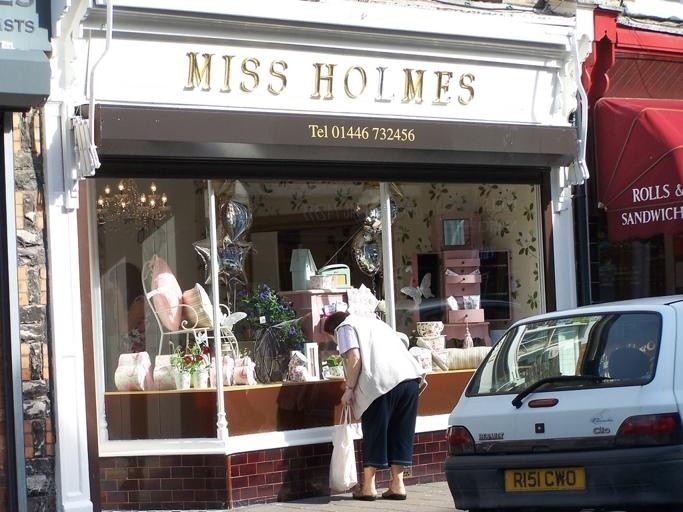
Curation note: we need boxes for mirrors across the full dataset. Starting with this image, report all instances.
[443,219,471,247]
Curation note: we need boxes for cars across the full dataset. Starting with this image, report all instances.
[444,293,683,511]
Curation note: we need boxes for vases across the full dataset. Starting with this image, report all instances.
[175,372,191,389]
[193,370,209,388]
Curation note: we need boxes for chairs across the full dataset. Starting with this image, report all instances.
[141,260,239,359]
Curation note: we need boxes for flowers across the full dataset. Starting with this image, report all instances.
[192,346,213,368]
[170,345,194,372]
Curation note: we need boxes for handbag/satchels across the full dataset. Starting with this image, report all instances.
[329,423,358,492]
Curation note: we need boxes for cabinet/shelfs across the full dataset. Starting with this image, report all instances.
[442,249,485,324]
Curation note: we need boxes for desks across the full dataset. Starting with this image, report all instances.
[443,323,492,346]
[277,290,348,345]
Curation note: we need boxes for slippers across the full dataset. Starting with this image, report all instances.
[352,491,374,499]
[381,489,406,499]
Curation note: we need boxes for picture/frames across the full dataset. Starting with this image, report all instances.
[304,343,320,380]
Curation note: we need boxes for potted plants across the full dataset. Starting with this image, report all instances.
[323,355,345,379]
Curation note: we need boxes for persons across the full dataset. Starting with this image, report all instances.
[323,312,426,499]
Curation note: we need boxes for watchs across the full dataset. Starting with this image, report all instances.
[344,385,356,390]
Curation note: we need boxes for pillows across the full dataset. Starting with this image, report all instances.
[184,283,213,326]
[151,256,184,332]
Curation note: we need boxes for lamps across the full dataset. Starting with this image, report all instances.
[97,178,172,228]
